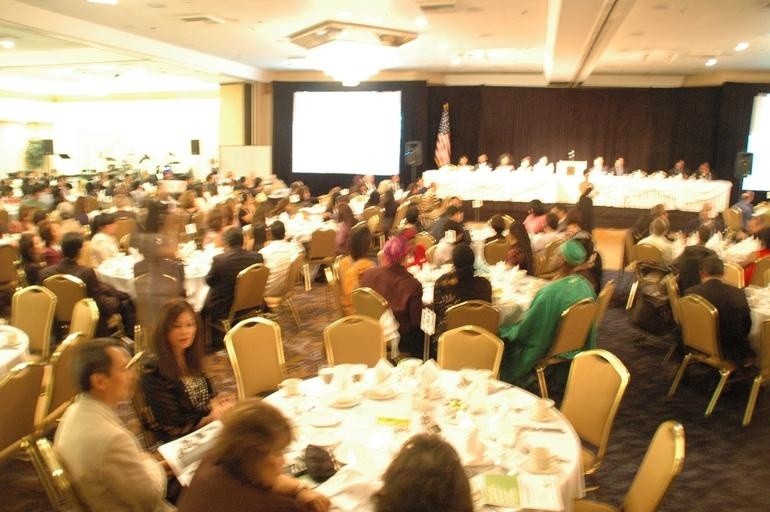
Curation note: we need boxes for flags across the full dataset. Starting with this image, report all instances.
[434,103,452,169]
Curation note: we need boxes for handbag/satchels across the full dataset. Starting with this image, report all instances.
[631,285,672,337]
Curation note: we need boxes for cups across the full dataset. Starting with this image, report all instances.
[282,357,555,472]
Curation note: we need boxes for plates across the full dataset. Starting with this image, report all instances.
[282,383,553,463]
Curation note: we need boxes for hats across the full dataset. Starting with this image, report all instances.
[560,240,587,266]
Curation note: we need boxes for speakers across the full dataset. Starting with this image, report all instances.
[42,139,54,156]
[191,140,199,155]
[404,141,423,166]
[736,151,753,176]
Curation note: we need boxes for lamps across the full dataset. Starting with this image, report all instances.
[288,19,420,88]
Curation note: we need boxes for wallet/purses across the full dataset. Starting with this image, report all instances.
[305,445,336,482]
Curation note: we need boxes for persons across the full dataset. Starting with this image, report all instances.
[0,155,770,512]
